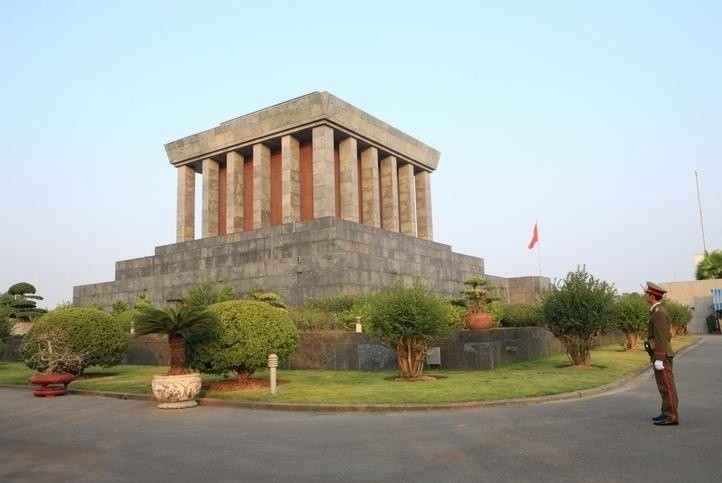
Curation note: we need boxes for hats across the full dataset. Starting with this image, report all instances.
[646,281,667,298]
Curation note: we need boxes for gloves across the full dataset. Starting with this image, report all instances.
[654,360,664,370]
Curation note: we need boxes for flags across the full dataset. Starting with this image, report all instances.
[528,223,537,250]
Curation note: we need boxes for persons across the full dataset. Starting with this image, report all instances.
[643,281,681,426]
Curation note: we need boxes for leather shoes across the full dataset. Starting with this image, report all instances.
[653,414,679,425]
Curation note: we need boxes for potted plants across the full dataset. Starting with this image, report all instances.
[131,302,219,408]
[459,276,496,329]
